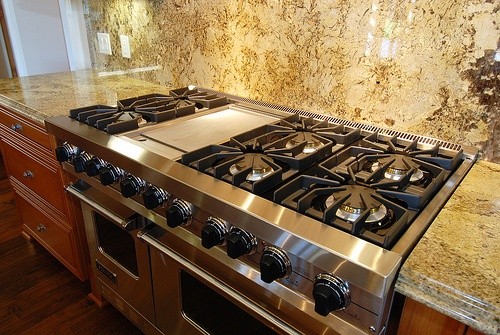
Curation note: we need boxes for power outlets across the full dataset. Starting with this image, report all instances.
[120,34,132,60]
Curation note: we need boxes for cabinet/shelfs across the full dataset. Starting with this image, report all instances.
[391,159,500,335]
[0,68,179,286]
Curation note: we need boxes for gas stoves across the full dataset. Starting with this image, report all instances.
[43,85,478,334]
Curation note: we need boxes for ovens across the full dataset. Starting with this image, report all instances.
[63,171,342,334]
